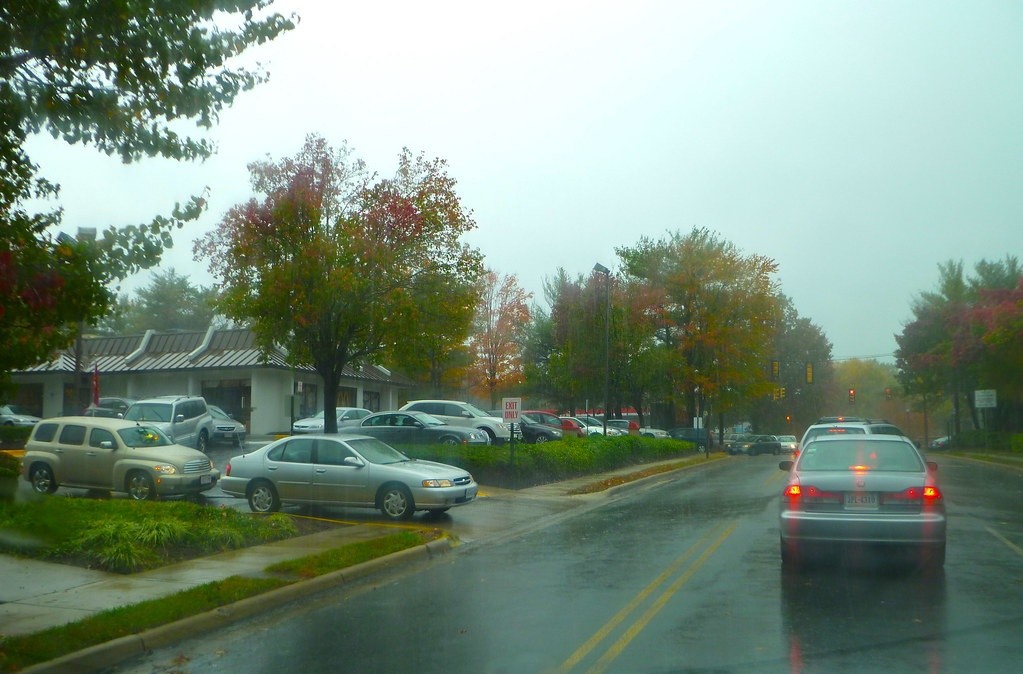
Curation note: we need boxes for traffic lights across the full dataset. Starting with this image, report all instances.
[786,416,792,423]
[773,388,781,400]
[885,387,896,401]
[780,387,786,398]
[849,389,857,404]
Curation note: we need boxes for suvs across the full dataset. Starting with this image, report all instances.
[668,425,718,451]
[390,399,524,444]
[122,395,217,453]
[794,416,921,462]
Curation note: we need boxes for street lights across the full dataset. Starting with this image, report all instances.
[592,263,611,438]
[51,225,100,415]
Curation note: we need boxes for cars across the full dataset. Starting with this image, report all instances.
[205,404,251,447]
[336,410,490,448]
[714,432,742,450]
[549,413,672,439]
[775,434,951,570]
[523,410,587,438]
[777,434,799,452]
[22,416,221,503]
[727,434,784,455]
[0,422,35,477]
[218,433,478,515]
[0,401,43,425]
[490,409,567,442]
[85,397,134,419]
[928,433,954,450]
[292,407,377,434]
